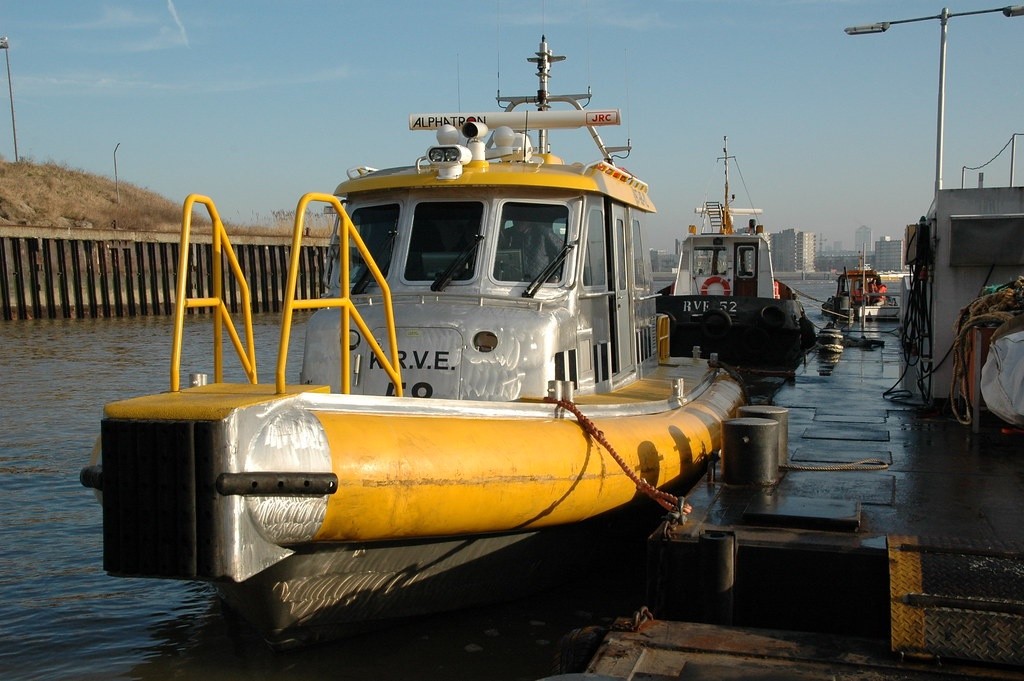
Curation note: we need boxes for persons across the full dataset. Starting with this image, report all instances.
[505,219,566,283]
[851,278,887,306]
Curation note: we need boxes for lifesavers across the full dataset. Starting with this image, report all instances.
[774,282,778,299]
[700,277,731,295]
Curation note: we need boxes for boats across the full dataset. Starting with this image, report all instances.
[78,32,753,648]
[649,137,811,365]
[820,250,902,318]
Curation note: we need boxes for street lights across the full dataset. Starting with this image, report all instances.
[841,0,1023,198]
